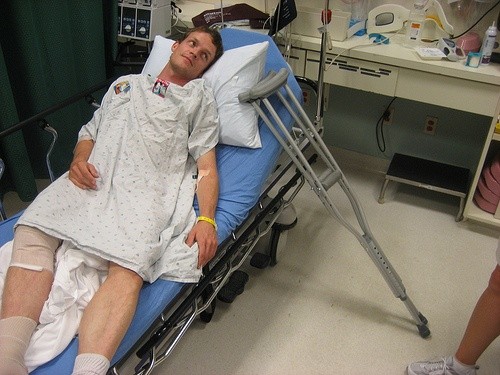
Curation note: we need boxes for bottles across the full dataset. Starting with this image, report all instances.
[480,20,497,65]
[404,2,425,49]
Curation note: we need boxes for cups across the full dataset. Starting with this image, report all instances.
[465,51,481,67]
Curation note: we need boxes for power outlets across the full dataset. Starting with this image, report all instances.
[383,108,394,125]
[423,117,438,136]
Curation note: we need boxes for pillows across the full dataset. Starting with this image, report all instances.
[135,36,269,149]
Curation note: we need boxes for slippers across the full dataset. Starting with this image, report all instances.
[217,270,249,304]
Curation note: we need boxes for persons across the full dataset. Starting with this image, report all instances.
[0,25,225,375]
[407,241,500,375]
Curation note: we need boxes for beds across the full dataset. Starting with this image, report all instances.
[0,27,326,375]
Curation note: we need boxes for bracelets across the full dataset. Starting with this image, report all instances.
[196,216,217,228]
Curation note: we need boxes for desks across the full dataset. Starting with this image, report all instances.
[169,0,500,228]
[378,153,471,221]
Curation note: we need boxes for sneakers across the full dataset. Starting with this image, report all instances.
[405,356,480,375]
